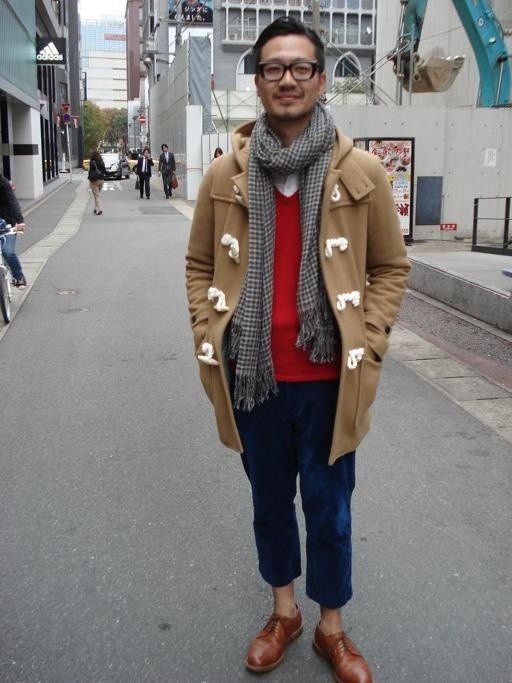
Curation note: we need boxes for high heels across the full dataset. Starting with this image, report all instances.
[96,210,102,214]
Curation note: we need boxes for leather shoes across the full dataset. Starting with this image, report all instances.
[312,618,372,683]
[169,191,172,196]
[166,195,169,198]
[246,603,304,672]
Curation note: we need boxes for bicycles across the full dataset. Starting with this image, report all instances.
[0,218,24,325]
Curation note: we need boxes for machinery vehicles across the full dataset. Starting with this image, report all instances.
[387,0,511,109]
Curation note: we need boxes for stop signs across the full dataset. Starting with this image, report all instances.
[137,115,146,123]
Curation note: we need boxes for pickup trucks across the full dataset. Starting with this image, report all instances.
[80,153,138,174]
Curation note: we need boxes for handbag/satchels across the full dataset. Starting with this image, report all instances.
[171,173,178,188]
[92,159,103,179]
[135,174,141,189]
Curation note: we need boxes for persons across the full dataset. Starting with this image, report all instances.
[136,148,154,199]
[87,150,107,215]
[0,172,27,290]
[185,17,412,683]
[123,148,142,162]
[157,143,176,197]
[213,147,223,159]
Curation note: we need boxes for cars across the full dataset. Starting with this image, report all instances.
[98,151,131,180]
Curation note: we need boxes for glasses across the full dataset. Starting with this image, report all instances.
[257,61,321,81]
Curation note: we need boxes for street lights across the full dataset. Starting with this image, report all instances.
[131,114,137,153]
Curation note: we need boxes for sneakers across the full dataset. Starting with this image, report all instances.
[18,272,27,289]
